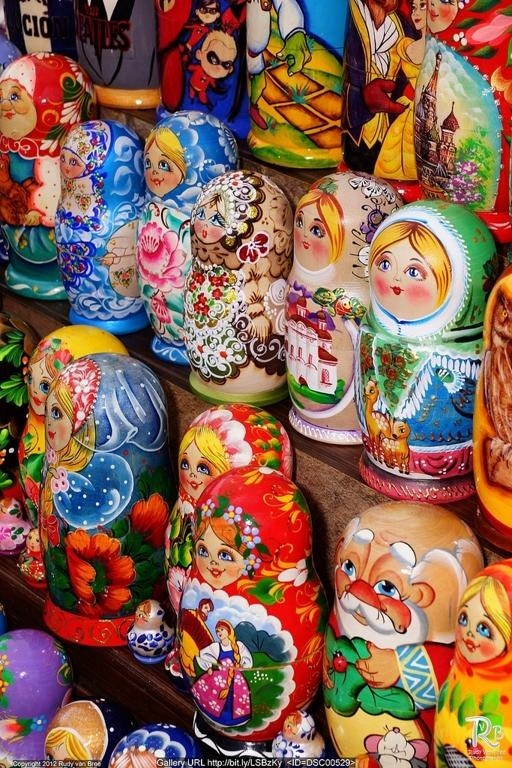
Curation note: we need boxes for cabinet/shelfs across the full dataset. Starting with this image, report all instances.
[1,77,512,766]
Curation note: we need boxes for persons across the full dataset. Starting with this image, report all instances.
[0,0,512,768]
